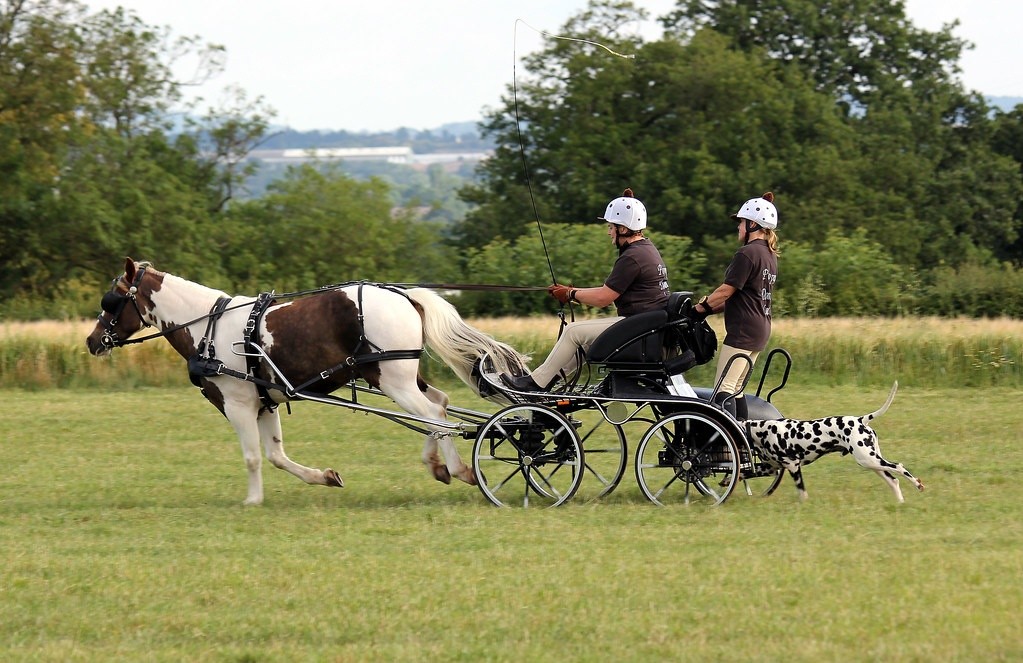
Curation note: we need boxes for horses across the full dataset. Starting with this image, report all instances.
[84,256,533,508]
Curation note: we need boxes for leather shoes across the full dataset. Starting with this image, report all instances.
[499,373,561,403]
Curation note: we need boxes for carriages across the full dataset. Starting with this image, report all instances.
[82,257,794,510]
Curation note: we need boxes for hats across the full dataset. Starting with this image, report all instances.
[730,192,777,229]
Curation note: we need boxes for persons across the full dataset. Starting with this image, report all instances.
[498,189,671,400]
[693,192,781,421]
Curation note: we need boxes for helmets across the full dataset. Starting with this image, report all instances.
[597,188,647,231]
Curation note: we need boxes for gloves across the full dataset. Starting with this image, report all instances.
[686,306,709,324]
[548,283,578,303]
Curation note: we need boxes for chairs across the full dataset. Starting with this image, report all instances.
[586,291,698,396]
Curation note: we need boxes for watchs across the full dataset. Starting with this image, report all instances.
[570,290,581,306]
[699,296,712,311]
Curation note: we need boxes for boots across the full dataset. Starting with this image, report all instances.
[713,392,735,461]
[735,395,751,461]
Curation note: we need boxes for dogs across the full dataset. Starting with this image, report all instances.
[733,381,925,505]
